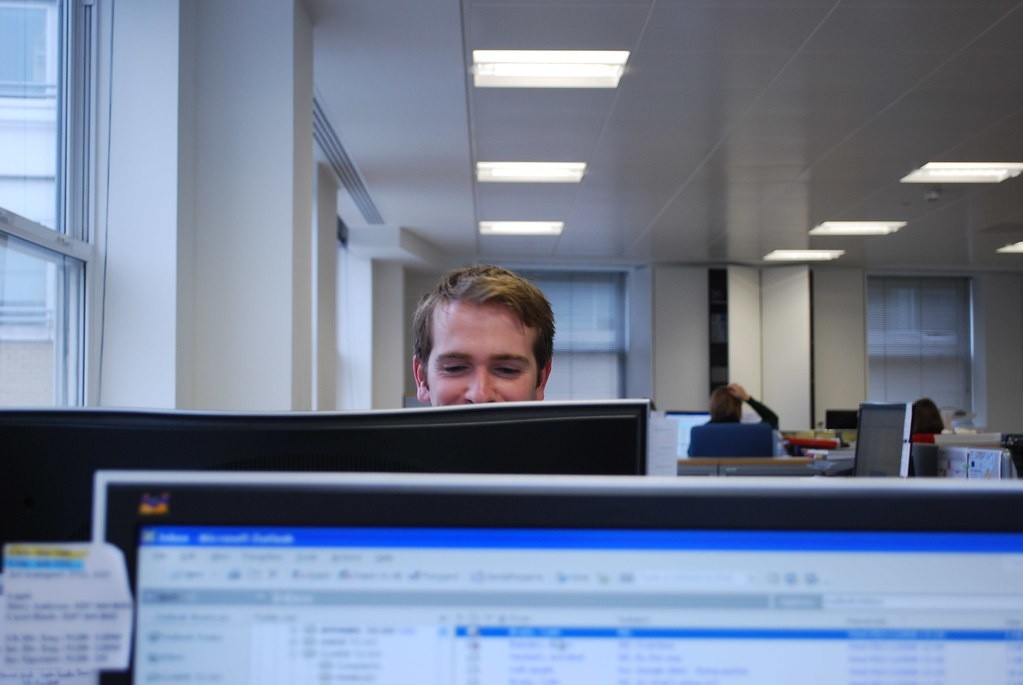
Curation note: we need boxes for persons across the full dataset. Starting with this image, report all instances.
[410,265,556,408]
[688,383,778,455]
[910,397,945,443]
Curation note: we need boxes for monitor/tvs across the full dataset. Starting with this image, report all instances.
[0,401,1023,685]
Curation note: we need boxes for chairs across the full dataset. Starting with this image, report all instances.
[690,422,775,460]
